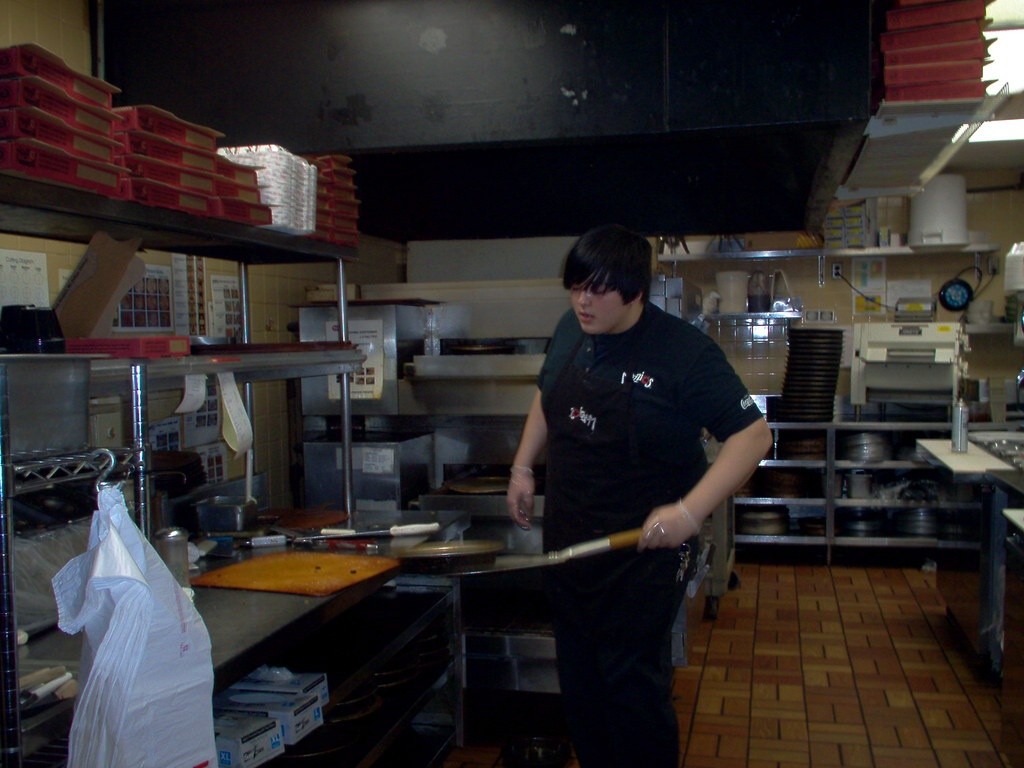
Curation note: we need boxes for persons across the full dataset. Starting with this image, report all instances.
[506,224,778,768]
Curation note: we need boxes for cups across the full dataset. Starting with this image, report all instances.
[715,270,748,313]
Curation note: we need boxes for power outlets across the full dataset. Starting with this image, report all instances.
[832,263,842,278]
[988,257,999,273]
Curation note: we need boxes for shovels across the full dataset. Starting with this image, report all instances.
[399,526,649,578]
[270,523,380,549]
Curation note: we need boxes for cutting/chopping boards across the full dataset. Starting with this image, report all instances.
[190,550,401,597]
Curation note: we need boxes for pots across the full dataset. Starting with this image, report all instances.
[402,541,505,575]
[908,173,969,246]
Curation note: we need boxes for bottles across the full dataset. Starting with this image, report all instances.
[153,526,193,604]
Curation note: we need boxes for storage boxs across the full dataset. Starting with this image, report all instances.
[213,688,324,744]
[880,0,996,101]
[0,42,362,358]
[213,709,285,768]
[229,665,329,707]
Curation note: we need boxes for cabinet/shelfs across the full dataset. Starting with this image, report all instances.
[1,174,1024,768]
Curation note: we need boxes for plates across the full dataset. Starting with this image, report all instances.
[776,324,843,423]
